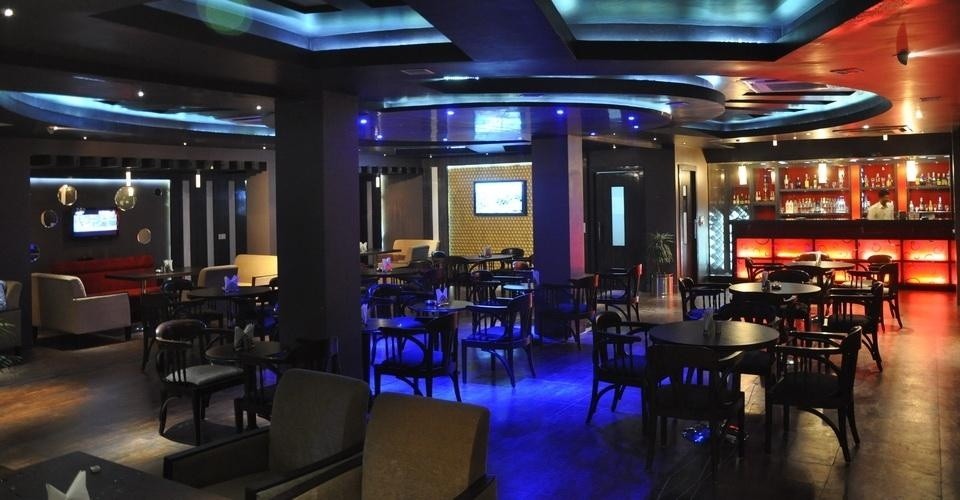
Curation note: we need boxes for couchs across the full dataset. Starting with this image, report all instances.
[232,254,278,287]
[161,368,373,500]
[29,272,131,347]
[393,238,439,257]
[49,255,162,299]
[271,390,498,500]
[181,265,238,315]
[0,278,23,353]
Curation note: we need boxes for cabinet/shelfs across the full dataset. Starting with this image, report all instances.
[708,154,956,280]
[727,219,959,291]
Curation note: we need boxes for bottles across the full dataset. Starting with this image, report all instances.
[942,173,948,185]
[875,173,881,188]
[804,173,810,189]
[784,174,789,189]
[812,174,818,190]
[886,174,894,188]
[795,176,802,189]
[937,173,941,186]
[938,196,943,211]
[919,174,926,186]
[927,173,931,186]
[929,200,933,211]
[731,191,751,205]
[790,181,794,189]
[863,174,870,187]
[783,196,848,214]
[881,174,885,189]
[919,197,925,211]
[870,174,876,188]
[931,172,937,186]
[909,200,915,212]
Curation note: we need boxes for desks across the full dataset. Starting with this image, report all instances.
[729,282,822,304]
[502,282,538,292]
[783,259,856,322]
[104,266,200,324]
[407,299,473,361]
[365,268,419,285]
[361,247,401,269]
[463,255,513,264]
[0,450,237,500]
[204,341,287,432]
[649,320,780,357]
[360,318,389,382]
[186,285,276,328]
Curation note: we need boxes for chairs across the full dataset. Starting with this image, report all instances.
[461,293,537,389]
[373,312,462,402]
[293,336,341,374]
[787,265,825,285]
[597,264,642,323]
[678,277,728,321]
[768,269,809,284]
[765,325,862,462]
[585,311,668,440]
[821,281,883,373]
[795,254,834,261]
[877,263,904,333]
[154,318,245,447]
[139,293,224,374]
[160,279,223,348]
[645,345,745,487]
[537,273,599,354]
[432,255,468,300]
[847,255,891,288]
[714,300,776,383]
[744,258,784,280]
[501,248,524,271]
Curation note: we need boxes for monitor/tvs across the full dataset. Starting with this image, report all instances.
[471,179,527,217]
[70,207,121,241]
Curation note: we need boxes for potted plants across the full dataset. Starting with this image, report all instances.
[645,231,674,297]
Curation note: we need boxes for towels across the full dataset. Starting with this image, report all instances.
[436,287,447,304]
[225,275,238,292]
[45,470,90,500]
[233,324,255,350]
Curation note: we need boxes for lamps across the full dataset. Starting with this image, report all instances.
[897,50,916,66]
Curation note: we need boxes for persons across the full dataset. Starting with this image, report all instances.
[866,189,895,220]
